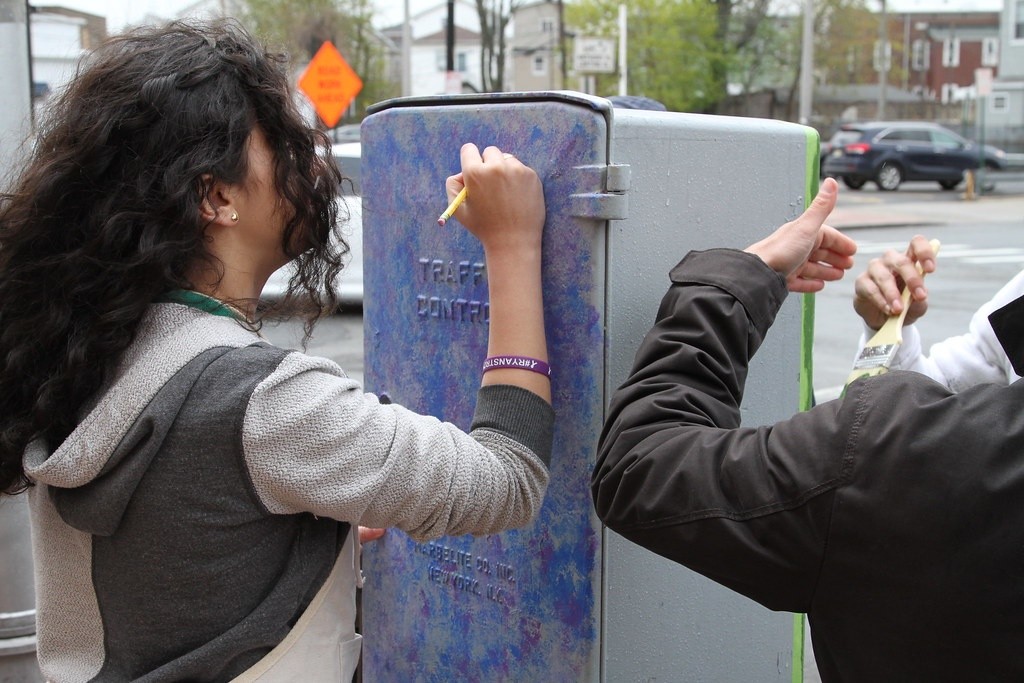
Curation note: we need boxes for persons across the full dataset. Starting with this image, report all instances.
[0,18,556,683]
[591,176,1024,683]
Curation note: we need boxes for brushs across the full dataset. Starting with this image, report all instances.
[848,238,941,385]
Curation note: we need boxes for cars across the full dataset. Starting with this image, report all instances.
[822,120,1008,193]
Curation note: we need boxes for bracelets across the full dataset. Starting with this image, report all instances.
[482,355,553,379]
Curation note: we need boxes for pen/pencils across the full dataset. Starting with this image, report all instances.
[437,185,467,227]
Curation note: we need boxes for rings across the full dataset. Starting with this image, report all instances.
[504,154,514,159]
